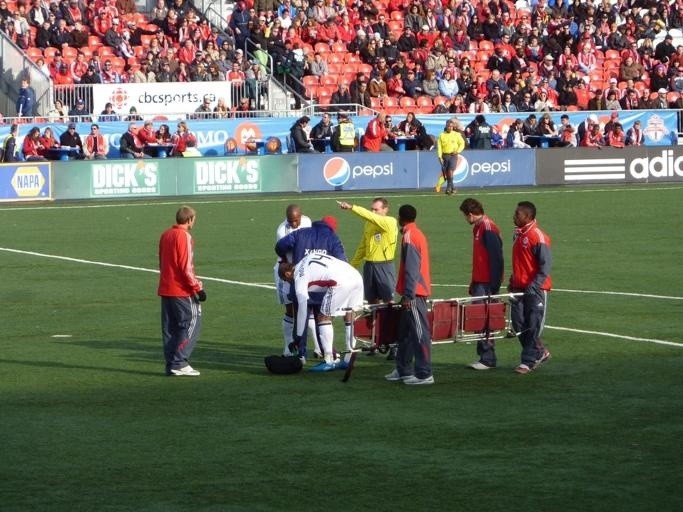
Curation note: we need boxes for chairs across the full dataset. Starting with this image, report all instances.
[1,1,683,162]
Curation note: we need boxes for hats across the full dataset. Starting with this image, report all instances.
[322,217,338,231]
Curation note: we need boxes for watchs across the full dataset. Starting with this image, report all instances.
[274,215,347,364]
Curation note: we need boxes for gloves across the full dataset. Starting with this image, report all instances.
[198,290,206,302]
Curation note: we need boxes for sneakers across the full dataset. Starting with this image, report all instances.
[171,365,201,376]
[403,375,434,385]
[467,349,551,374]
[385,369,413,381]
[367,348,378,356]
[387,348,398,360]
[308,348,349,373]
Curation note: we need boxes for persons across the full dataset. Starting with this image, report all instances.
[507,201,552,375]
[466,113,644,151]
[277,252,364,373]
[119,120,197,159]
[436,120,465,192]
[3,123,107,162]
[0,0,272,123]
[156,206,206,376]
[274,204,340,359]
[290,112,433,153]
[252,0,683,115]
[383,204,435,386]
[458,197,504,371]
[336,196,398,306]
